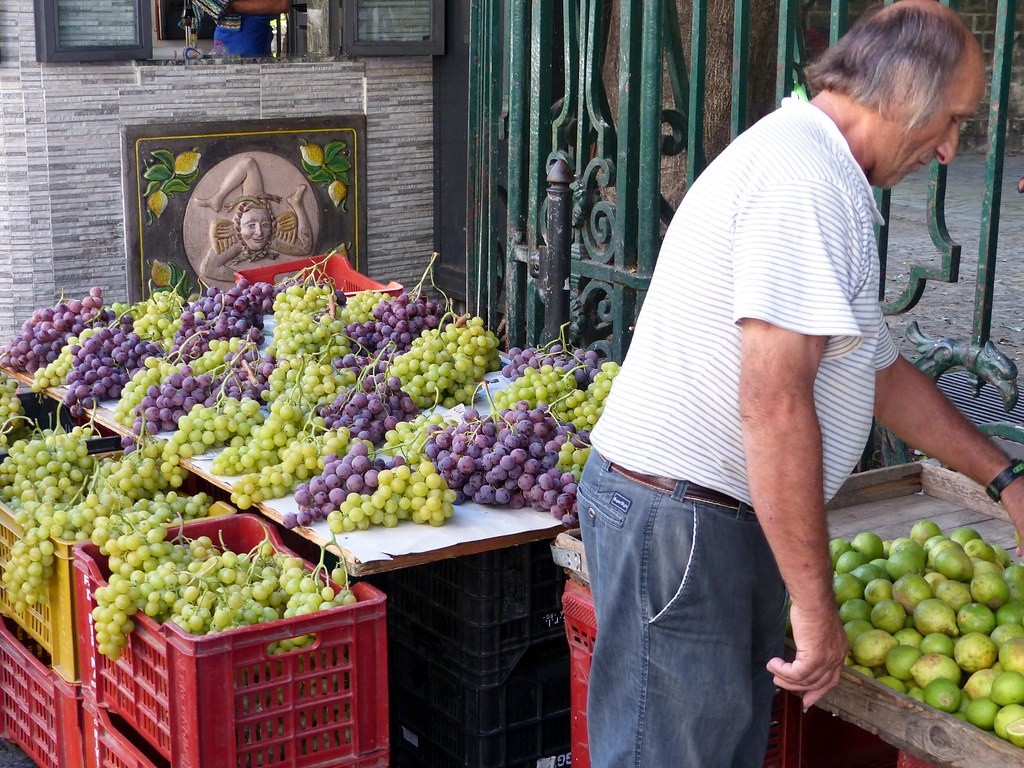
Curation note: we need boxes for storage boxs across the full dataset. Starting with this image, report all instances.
[0,252,938,768]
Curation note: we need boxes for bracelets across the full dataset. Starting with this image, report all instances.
[985,460,1024,503]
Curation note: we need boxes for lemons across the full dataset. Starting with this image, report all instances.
[828,518,1024,748]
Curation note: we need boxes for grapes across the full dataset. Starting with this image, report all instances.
[0,242,621,767]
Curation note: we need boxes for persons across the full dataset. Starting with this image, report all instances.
[191,0,294,57]
[575,1,1023,767]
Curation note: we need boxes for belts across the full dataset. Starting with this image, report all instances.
[599,451,756,514]
